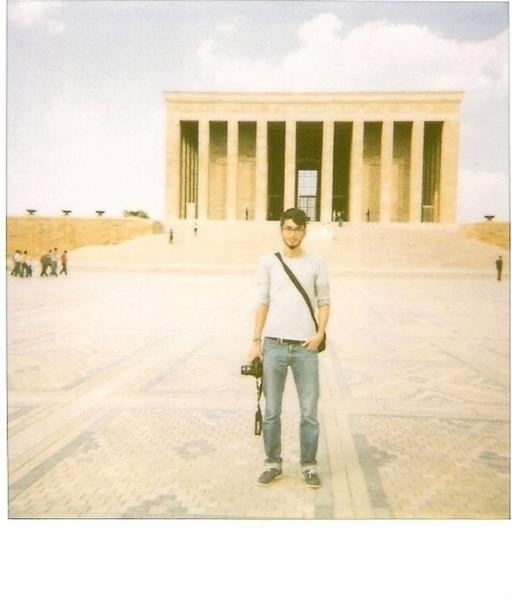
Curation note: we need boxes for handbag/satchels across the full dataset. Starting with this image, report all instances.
[317,331,325,352]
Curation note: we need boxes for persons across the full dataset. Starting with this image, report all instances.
[245,205,333,491]
[494,255,504,282]
[10,247,70,278]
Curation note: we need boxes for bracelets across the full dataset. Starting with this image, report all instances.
[251,337,262,344]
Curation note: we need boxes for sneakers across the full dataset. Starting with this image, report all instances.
[258,467,283,486]
[300,470,320,487]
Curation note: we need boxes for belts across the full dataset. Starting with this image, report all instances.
[266,337,305,344]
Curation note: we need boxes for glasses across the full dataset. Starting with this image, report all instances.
[281,226,305,231]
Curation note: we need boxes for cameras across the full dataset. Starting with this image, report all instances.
[241,358,263,378]
